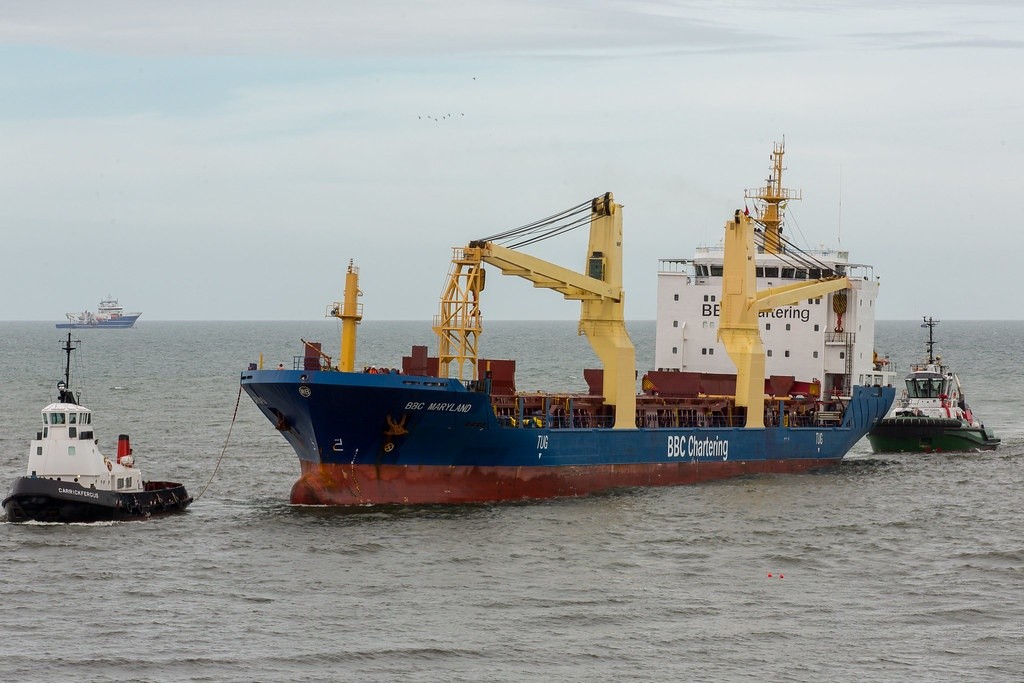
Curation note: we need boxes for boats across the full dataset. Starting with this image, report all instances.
[55,292,143,328]
[237,136,895,505]
[1,331,194,524]
[864,316,1002,453]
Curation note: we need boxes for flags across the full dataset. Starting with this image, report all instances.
[744,206,749,216]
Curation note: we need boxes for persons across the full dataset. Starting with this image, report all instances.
[575,408,591,428]
[362,366,400,375]
[937,383,943,394]
[922,381,928,397]
[71,417,76,423]
[278,364,283,370]
[795,408,815,427]
